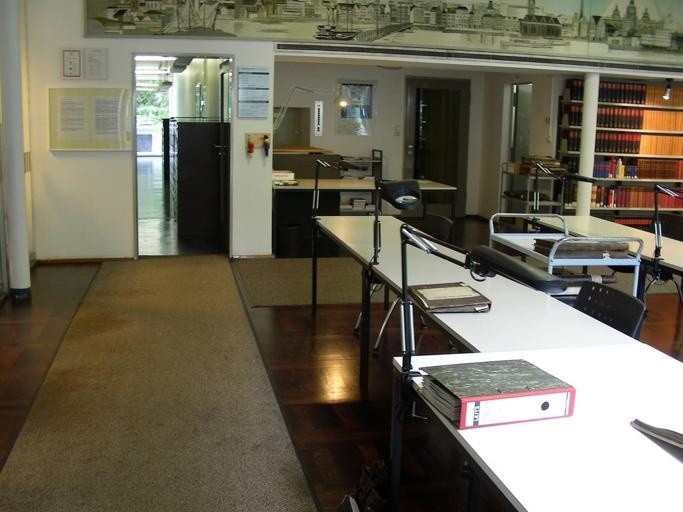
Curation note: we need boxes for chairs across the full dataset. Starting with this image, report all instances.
[574,280,647,339]
[355,216,454,350]
[375,180,427,216]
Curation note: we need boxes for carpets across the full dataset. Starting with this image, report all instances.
[0,255,322,511]
[232,256,397,308]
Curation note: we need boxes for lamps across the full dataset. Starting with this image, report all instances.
[662,79,675,100]
[275,86,351,135]
[158,56,194,92]
[396,222,569,510]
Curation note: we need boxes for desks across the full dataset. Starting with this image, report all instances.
[312,214,642,402]
[390,343,682,510]
[521,214,682,299]
[273,178,458,228]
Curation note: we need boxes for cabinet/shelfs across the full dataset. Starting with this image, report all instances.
[163,118,226,237]
[488,213,644,296]
[554,80,683,211]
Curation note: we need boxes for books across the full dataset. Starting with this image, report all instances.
[340,197,375,210]
[554,77,683,232]
[272,170,295,180]
[534,232,631,261]
